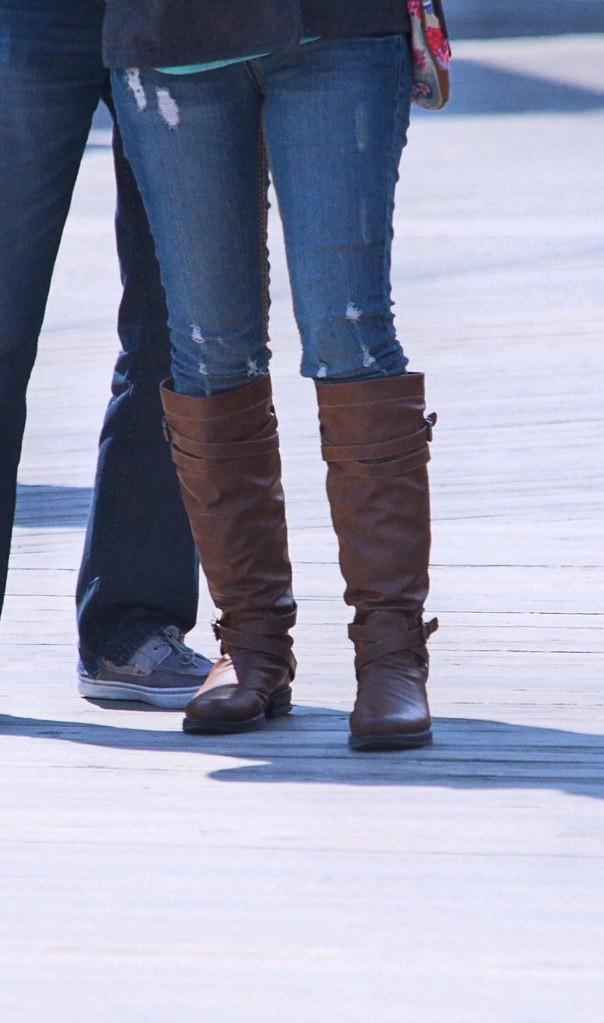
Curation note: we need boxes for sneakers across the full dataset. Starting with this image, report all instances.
[76,627,216,710]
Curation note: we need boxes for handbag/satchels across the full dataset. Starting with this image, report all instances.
[406,0,451,110]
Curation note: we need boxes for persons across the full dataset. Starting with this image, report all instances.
[0,0,214,708]
[102,0,438,747]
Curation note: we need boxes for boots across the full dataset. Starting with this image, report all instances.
[315,371,439,749]
[159,368,297,734]
